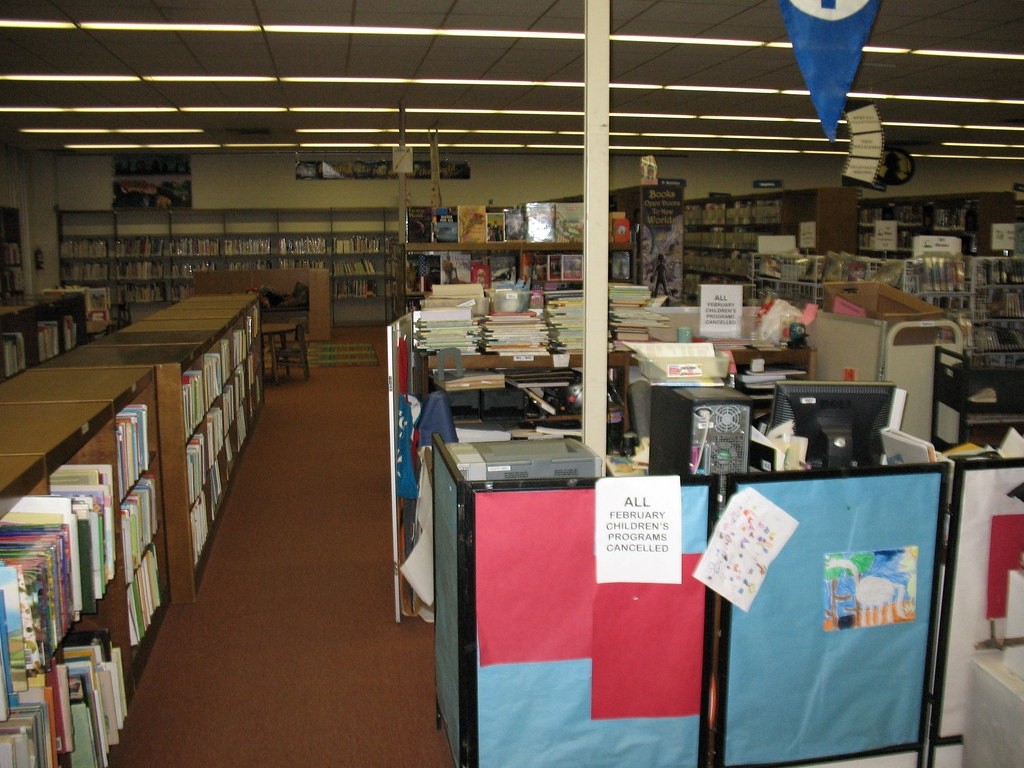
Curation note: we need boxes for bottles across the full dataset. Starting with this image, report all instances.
[739,306,761,341]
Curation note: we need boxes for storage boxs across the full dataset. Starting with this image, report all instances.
[820,281,946,345]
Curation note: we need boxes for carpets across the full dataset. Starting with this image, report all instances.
[264,342,377,367]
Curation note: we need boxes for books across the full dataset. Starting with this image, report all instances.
[424,362,576,392]
[752,250,1024,357]
[332,280,377,298]
[61,236,396,257]
[523,388,555,415]
[2,243,24,298]
[85,286,108,324]
[65,261,398,281]
[182,305,261,569]
[408,251,639,293]
[452,409,583,447]
[855,200,970,251]
[0,309,77,379]
[683,200,784,295]
[0,402,162,767]
[880,427,936,465]
[413,283,678,356]
[109,281,194,303]
[406,204,631,242]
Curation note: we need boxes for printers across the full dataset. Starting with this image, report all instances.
[445,438,603,482]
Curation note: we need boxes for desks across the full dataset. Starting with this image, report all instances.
[260,322,297,381]
[86,319,110,339]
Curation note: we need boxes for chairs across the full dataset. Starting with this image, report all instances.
[274,323,308,381]
[109,300,130,334]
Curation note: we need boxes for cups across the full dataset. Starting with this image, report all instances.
[677,327,693,343]
[779,312,797,344]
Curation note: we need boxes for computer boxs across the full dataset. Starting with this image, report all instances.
[649,385,754,475]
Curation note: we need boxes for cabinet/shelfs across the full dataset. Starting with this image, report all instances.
[0,185,1024,767]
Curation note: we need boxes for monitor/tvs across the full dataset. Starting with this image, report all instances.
[764,379,896,469]
